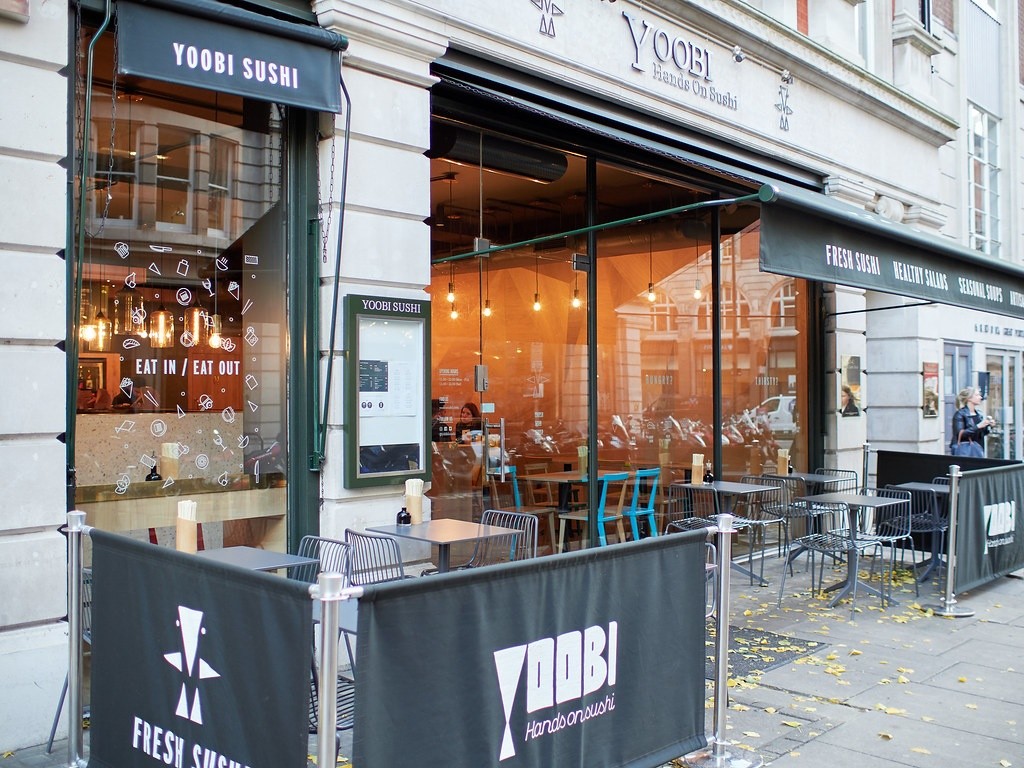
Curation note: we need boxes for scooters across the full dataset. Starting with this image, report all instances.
[514,408,789,472]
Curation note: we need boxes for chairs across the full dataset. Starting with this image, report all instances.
[45,565,92,755]
[289,461,953,768]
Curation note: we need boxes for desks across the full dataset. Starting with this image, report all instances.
[192,546,321,570]
[674,477,781,584]
[507,470,637,552]
[795,489,912,609]
[893,483,959,584]
[364,518,524,576]
[765,472,857,567]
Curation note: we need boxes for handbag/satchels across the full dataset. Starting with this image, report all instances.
[950,429,983,457]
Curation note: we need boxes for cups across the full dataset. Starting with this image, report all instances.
[692,463,703,484]
[160,455,178,479]
[578,457,587,473]
[778,456,788,475]
[405,496,422,524]
[176,516,197,554]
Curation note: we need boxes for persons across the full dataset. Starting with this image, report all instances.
[949,388,995,455]
[111,378,146,409]
[841,385,858,417]
[431,399,452,442]
[456,403,489,444]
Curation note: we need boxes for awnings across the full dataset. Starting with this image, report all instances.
[115,0,350,115]
[432,70,1024,319]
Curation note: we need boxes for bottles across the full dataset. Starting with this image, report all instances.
[703,463,714,484]
[145,450,162,481]
[788,455,793,474]
[397,498,411,527]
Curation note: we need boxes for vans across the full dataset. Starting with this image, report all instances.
[640,394,732,428]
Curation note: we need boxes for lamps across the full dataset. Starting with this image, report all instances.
[83,92,222,353]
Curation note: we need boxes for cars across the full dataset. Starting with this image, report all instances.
[739,393,799,434]
